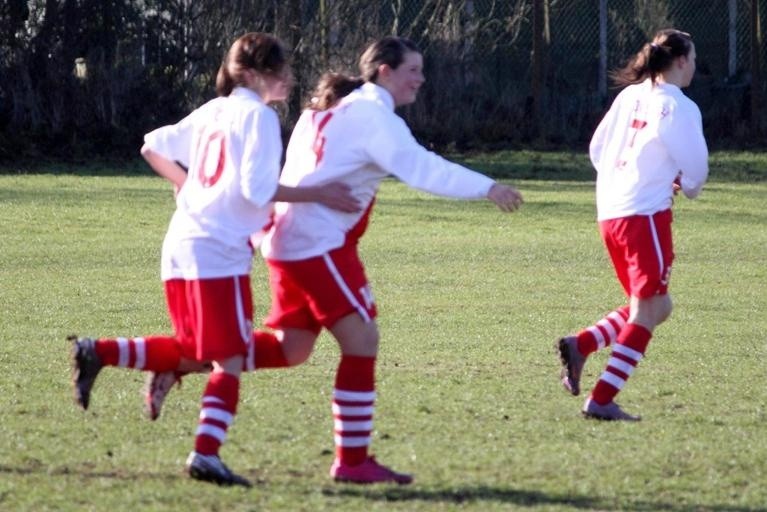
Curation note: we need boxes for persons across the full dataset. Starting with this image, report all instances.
[62,33,364,490]
[550,28,712,422]
[145,36,526,488]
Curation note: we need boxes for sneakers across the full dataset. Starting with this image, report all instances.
[144,371,178,421]
[558,334,586,396]
[330,457,413,484]
[583,397,640,422]
[69,337,103,408]
[184,451,250,487]
